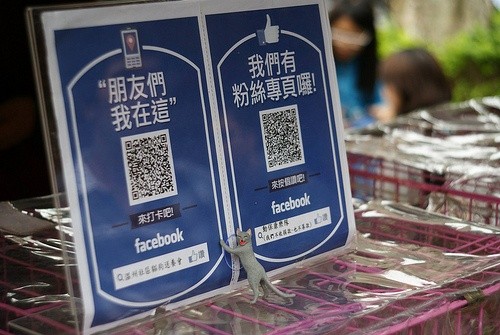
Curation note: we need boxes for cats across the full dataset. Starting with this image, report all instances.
[220,228,296,305]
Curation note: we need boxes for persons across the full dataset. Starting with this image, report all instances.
[0,0,54,202]
[328,1,455,123]
